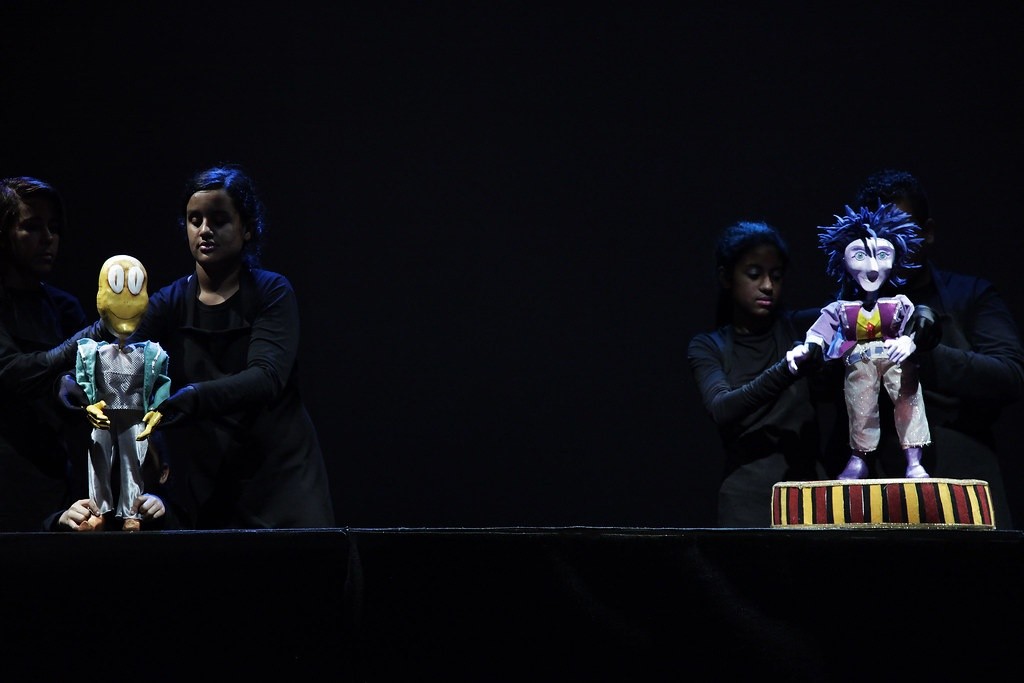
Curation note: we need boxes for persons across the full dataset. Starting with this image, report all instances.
[58,255,170,530]
[0,163,336,532]
[853,169,1024,525]
[786,204,931,479]
[688,222,822,529]
